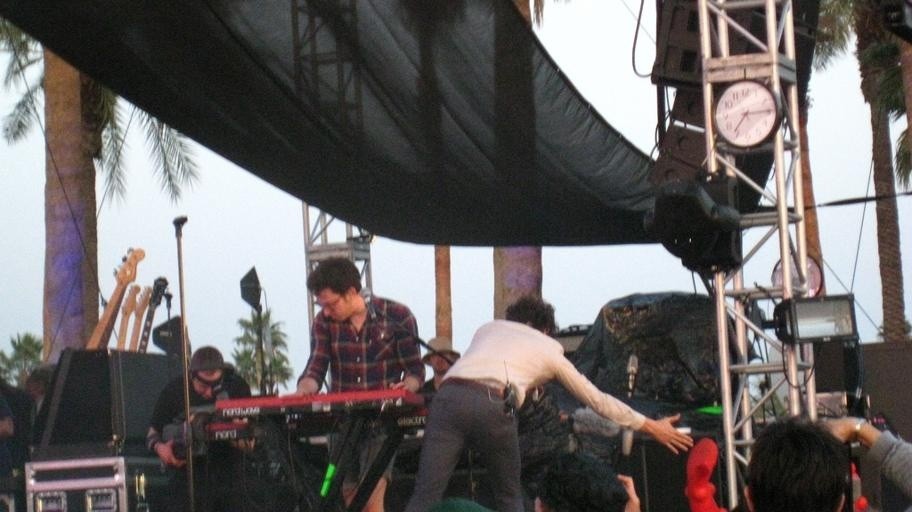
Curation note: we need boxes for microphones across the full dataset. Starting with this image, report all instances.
[626,354,639,398]
[360,288,377,320]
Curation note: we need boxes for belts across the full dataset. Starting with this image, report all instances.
[436,375,502,398]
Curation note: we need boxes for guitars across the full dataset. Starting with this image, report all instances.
[161,414,298,460]
[85,248,168,354]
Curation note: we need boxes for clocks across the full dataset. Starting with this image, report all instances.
[714,81,784,149]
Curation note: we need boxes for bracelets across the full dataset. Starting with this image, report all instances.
[853,417,867,439]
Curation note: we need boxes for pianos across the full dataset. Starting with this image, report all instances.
[215,390,427,419]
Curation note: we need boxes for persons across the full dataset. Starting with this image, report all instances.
[743,406,911,512]
[404,295,697,511]
[28,358,58,417]
[415,336,462,395]
[294,256,424,511]
[0,392,24,511]
[150,346,258,512]
[469,380,571,510]
[533,449,646,511]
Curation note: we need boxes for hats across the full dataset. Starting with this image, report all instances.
[187,347,236,376]
[420,335,460,367]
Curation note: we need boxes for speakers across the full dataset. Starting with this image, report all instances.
[638,432,725,512]
